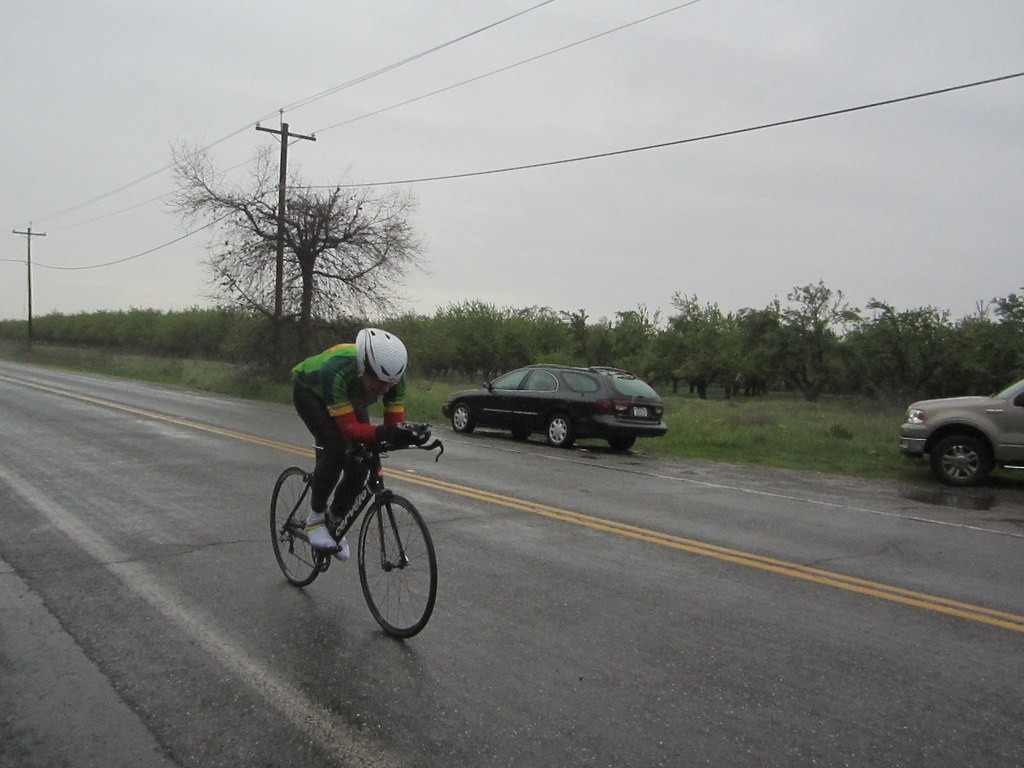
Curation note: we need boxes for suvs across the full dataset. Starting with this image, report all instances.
[442,364,667,452]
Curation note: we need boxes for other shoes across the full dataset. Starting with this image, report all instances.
[304,520,338,551]
[332,531,350,561]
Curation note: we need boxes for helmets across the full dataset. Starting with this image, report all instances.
[356,327,408,384]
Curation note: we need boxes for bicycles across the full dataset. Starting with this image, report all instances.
[269,424,448,638]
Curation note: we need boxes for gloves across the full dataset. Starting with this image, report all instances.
[376,422,432,446]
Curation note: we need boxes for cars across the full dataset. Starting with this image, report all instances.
[898,376,1024,486]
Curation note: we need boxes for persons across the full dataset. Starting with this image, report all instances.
[290,326,408,561]
